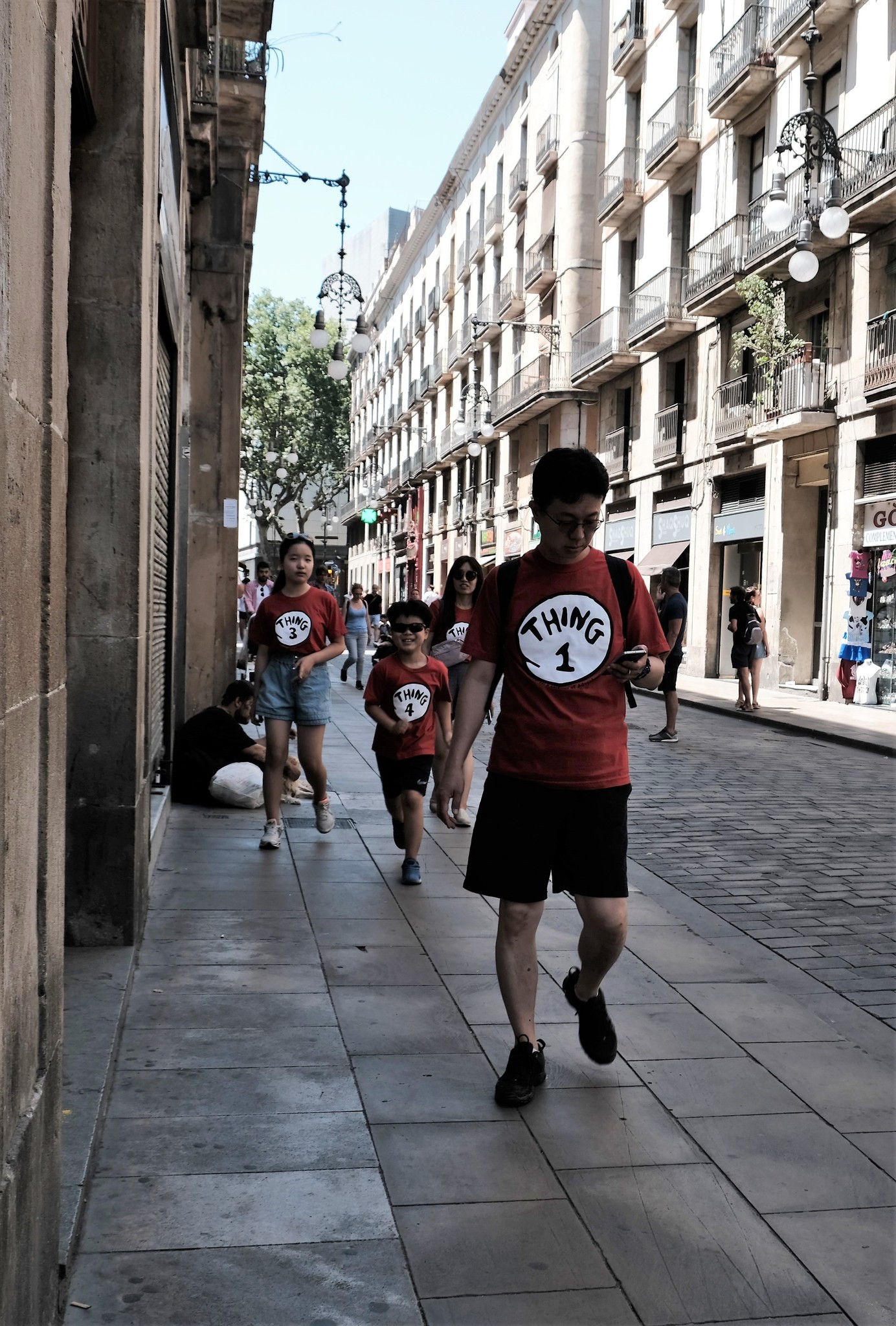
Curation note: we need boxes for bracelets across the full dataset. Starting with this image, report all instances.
[634,658,651,681]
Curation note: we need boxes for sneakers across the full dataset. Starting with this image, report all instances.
[392,818,406,849]
[649,728,678,742]
[495,1034,546,1108]
[259,819,284,848]
[401,858,422,885]
[562,966,617,1064]
[312,798,335,834]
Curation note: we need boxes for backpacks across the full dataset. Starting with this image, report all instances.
[732,604,763,645]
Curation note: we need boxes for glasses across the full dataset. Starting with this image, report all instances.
[543,508,605,533]
[261,587,264,597]
[392,623,426,633]
[453,569,477,581]
[283,532,314,544]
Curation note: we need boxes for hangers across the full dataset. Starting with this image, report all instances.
[853,544,870,554]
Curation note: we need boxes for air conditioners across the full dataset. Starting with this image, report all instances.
[779,362,826,414]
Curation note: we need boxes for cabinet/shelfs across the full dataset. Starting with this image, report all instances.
[873,556,896,707]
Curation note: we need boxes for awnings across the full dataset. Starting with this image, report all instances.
[477,555,496,568]
[608,550,635,561]
[635,541,690,576]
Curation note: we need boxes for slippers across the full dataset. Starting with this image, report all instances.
[736,705,754,711]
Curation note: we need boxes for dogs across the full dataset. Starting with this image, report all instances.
[279,754,331,805]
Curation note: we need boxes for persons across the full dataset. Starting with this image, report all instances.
[373,634,395,648]
[434,448,668,1106]
[423,556,487,826]
[377,614,391,638]
[250,532,348,850]
[409,589,420,600]
[309,565,337,600]
[363,584,383,646]
[362,600,455,883]
[727,586,771,712]
[237,560,275,642]
[340,583,373,691]
[173,680,301,802]
[421,584,444,607]
[648,567,687,742]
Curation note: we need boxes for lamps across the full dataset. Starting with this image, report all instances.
[454,316,559,457]
[760,0,847,282]
[240,396,300,519]
[248,170,371,380]
[362,422,428,508]
[320,470,367,530]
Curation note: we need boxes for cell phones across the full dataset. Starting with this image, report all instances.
[601,651,649,676]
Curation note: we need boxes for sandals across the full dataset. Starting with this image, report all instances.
[736,699,745,707]
[752,701,761,709]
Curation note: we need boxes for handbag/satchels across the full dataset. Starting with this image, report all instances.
[431,638,469,667]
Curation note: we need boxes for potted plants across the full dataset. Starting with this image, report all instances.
[726,273,814,420]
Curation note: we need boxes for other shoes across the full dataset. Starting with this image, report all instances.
[356,681,364,690]
[430,796,438,813]
[341,669,347,681]
[372,641,380,647]
[454,808,471,827]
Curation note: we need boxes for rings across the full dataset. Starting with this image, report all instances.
[628,668,632,675]
